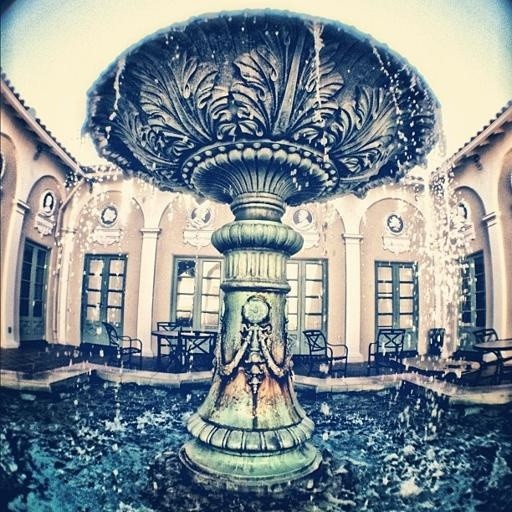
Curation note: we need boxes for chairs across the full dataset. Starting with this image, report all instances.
[100,321,143,371]
